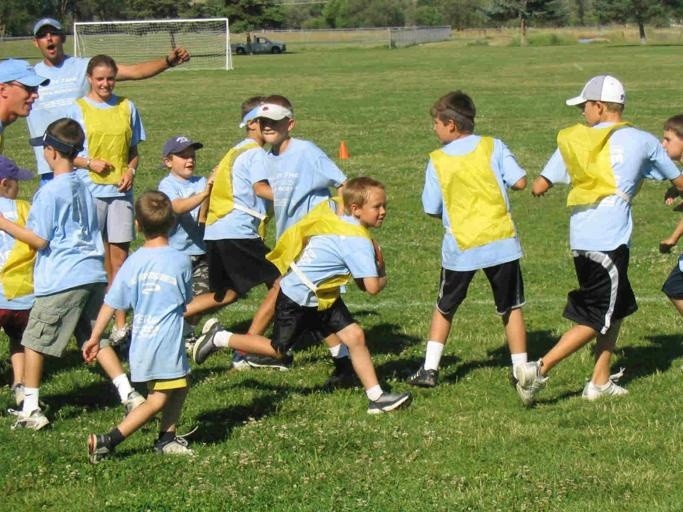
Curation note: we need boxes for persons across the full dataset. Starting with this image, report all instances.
[245,32,254,56]
[399,88,533,387]
[512,75,681,411]
[79,190,196,466]
[656,115,683,322]
[16,18,192,188]
[0,54,411,432]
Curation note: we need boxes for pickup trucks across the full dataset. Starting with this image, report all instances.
[231,33,289,54]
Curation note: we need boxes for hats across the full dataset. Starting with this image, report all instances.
[0,102,292,180]
[34,18,62,36]
[566,75,624,106]
[0,59,50,87]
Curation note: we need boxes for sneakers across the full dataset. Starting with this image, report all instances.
[88,432,112,465]
[152,426,200,455]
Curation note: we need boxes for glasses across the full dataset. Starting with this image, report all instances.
[37,29,59,38]
[10,83,38,92]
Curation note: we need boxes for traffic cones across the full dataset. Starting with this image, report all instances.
[335,142,348,158]
